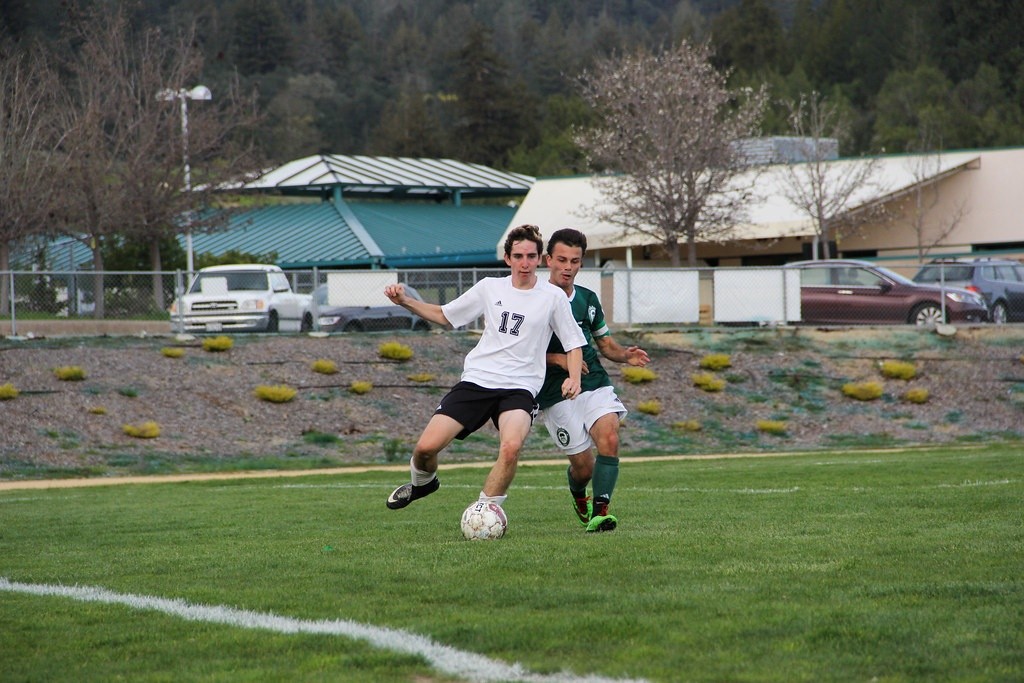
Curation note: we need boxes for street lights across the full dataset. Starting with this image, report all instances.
[153,85,212,286]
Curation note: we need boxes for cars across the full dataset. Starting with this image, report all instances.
[306,282,434,333]
[714,259,989,326]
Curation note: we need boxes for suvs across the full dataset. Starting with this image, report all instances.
[912,259,1024,324]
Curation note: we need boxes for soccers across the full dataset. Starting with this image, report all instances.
[460,500,509,543]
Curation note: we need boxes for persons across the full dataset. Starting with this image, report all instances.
[536,229,650,533]
[383,224,588,510]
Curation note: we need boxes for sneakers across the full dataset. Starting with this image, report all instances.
[585,515,618,534]
[569,486,594,527]
[386,476,440,509]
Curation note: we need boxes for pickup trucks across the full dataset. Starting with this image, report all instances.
[169,263,310,333]
